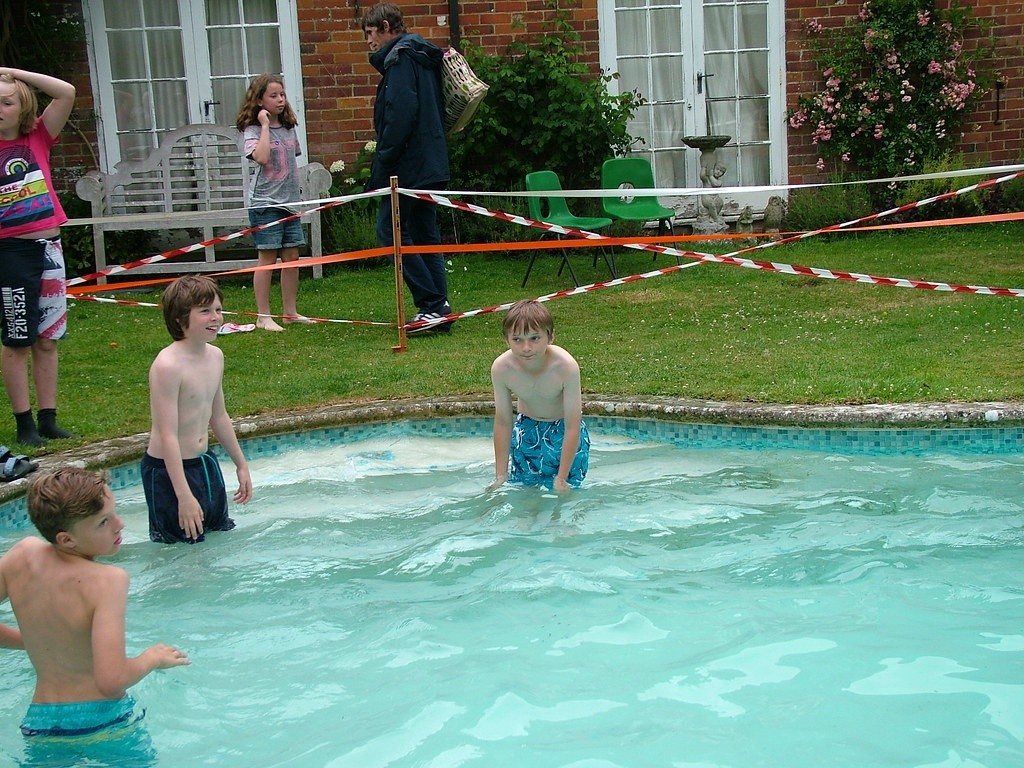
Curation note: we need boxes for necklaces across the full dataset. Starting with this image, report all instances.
[268,125,285,146]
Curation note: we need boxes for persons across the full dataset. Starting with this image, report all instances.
[0,464,195,768]
[0,64,80,448]
[233,72,322,334]
[136,277,253,548]
[482,297,592,539]
[358,0,456,338]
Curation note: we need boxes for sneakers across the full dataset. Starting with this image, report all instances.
[404,300,453,335]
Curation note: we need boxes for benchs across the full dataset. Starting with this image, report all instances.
[76,124,333,287]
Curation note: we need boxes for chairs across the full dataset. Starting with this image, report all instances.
[520,171,615,289]
[592,158,685,272]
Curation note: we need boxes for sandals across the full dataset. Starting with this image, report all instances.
[0,442,39,481]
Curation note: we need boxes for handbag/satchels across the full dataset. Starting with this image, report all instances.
[438,46,490,138]
